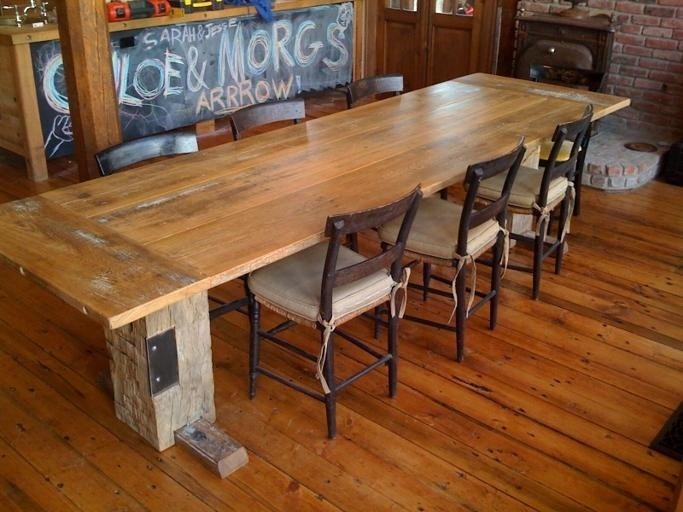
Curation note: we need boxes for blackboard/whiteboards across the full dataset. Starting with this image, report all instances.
[29,2,355,160]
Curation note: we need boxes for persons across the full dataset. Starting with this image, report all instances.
[462,1,473,16]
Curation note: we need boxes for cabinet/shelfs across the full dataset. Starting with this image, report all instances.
[513,12,615,94]
